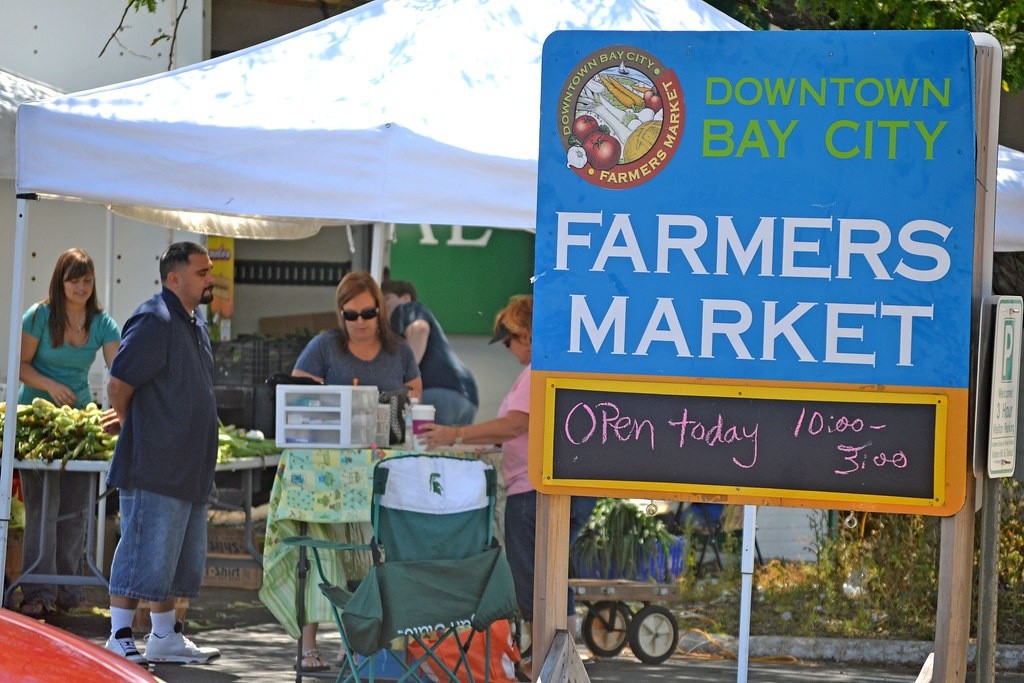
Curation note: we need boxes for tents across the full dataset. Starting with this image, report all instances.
[0,0,1024,683]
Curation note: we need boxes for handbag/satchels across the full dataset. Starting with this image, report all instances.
[406,619,521,683]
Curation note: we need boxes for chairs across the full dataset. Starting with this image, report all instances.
[298,453,519,683]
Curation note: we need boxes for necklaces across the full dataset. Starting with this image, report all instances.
[69,313,83,331]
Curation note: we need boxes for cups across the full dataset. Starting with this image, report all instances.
[411,404,437,450]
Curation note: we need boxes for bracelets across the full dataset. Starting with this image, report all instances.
[456,425,463,445]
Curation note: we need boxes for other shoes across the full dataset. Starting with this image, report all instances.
[21,603,43,618]
[46,608,74,626]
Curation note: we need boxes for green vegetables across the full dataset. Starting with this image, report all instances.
[0,396,267,474]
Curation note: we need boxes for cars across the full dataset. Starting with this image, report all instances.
[208,329,319,436]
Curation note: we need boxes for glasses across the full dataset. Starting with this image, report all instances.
[343,307,379,321]
[503,336,513,347]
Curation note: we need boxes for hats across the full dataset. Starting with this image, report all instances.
[489,323,510,344]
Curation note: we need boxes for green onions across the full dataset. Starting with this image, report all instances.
[567,499,676,582]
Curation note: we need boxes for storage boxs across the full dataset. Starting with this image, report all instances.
[572,538,686,581]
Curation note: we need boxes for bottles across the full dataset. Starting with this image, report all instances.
[404,398,420,449]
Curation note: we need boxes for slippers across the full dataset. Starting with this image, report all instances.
[335,647,358,667]
[300,649,330,671]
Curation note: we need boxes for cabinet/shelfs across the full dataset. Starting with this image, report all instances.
[275,383,379,450]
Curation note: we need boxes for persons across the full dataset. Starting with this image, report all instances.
[294,271,422,671]
[106,241,220,664]
[381,280,479,427]
[20,247,122,611]
[416,295,577,681]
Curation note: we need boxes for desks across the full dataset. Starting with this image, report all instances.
[0,453,284,611]
[258,442,508,683]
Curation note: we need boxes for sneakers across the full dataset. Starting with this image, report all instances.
[105,627,149,670]
[144,621,221,663]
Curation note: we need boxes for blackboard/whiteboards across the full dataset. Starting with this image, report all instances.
[544,378,949,509]
